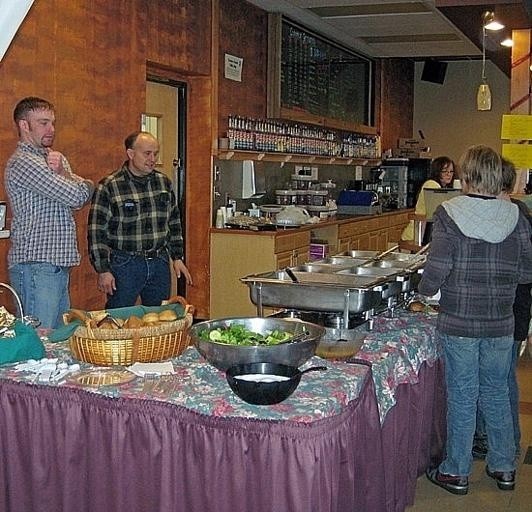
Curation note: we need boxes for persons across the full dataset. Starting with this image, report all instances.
[470,158,531,460]
[415,142,531,497]
[400,156,468,255]
[4,96,95,330]
[87,132,193,311]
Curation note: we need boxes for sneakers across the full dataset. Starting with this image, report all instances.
[471,445,486,460]
[426,466,469,495]
[485,465,517,490]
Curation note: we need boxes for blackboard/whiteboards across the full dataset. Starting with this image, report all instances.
[266,11,381,136]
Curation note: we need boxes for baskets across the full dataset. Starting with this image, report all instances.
[67,296,195,368]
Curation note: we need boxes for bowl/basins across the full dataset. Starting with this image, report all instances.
[315,331,365,361]
[189,318,325,369]
[227,361,302,406]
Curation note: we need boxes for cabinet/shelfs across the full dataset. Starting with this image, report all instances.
[207,223,311,325]
[312,210,417,255]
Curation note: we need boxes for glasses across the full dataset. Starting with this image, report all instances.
[441,171,455,176]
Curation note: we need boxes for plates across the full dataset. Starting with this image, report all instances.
[68,366,138,387]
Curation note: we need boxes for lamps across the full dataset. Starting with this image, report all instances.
[473,9,514,113]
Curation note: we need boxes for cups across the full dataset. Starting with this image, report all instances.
[218,138,229,149]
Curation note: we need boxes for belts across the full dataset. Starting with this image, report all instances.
[121,249,160,259]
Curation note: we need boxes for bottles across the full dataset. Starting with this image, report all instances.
[216,205,234,229]
[225,115,379,160]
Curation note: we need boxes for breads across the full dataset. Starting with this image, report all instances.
[86,310,178,329]
[408,302,425,312]
[77,371,135,385]
